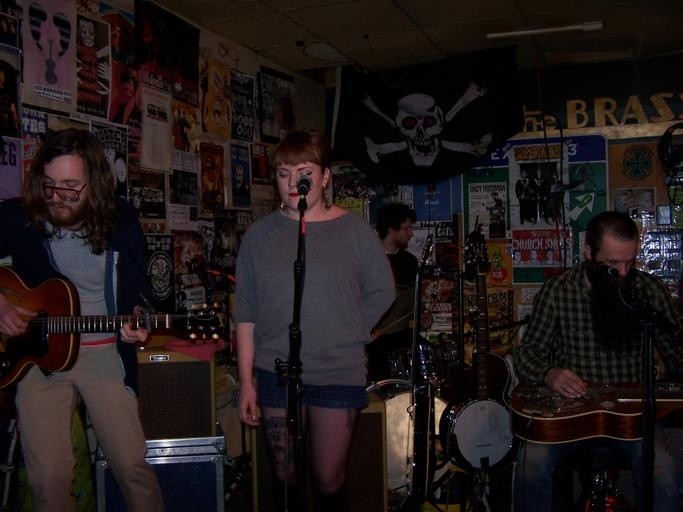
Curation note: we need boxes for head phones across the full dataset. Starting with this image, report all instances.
[656,122,683,170]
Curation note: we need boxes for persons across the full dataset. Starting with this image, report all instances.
[367,201,435,376]
[513,169,533,225]
[545,172,565,221]
[510,248,526,266]
[107,73,142,129]
[503,205,682,510]
[0,66,20,138]
[1,126,169,510]
[483,193,503,220]
[231,126,400,508]
[69,19,109,96]
[173,108,183,130]
[231,96,241,114]
[527,249,541,266]
[528,167,547,220]
[541,248,559,265]
[179,110,192,151]
[230,163,249,207]
[210,227,232,258]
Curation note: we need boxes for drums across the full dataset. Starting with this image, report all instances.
[383,344,434,388]
[359,379,455,496]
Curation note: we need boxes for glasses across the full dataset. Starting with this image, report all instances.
[41,182,87,203]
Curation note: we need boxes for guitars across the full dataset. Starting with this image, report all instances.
[438,221,520,472]
[509,381,683,445]
[0,264,226,390]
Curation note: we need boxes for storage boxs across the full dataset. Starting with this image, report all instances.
[95,436,226,512]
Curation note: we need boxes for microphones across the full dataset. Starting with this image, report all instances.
[582,260,618,276]
[420,233,433,259]
[296,174,314,195]
[480,456,491,495]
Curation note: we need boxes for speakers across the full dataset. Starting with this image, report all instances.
[305,392,389,512]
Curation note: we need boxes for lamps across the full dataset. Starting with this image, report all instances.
[481,19,603,40]
